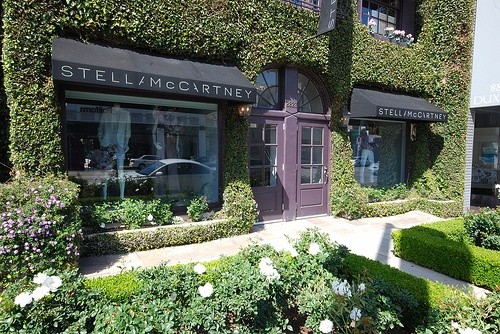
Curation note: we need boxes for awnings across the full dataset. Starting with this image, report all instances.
[51,35,257,107]
[348,84,450,123]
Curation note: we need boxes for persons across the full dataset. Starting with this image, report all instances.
[96,104,131,201]
[358,129,375,186]
[152,109,184,197]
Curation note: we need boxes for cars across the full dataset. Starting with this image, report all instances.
[129,154,162,170]
[116,158,216,202]
